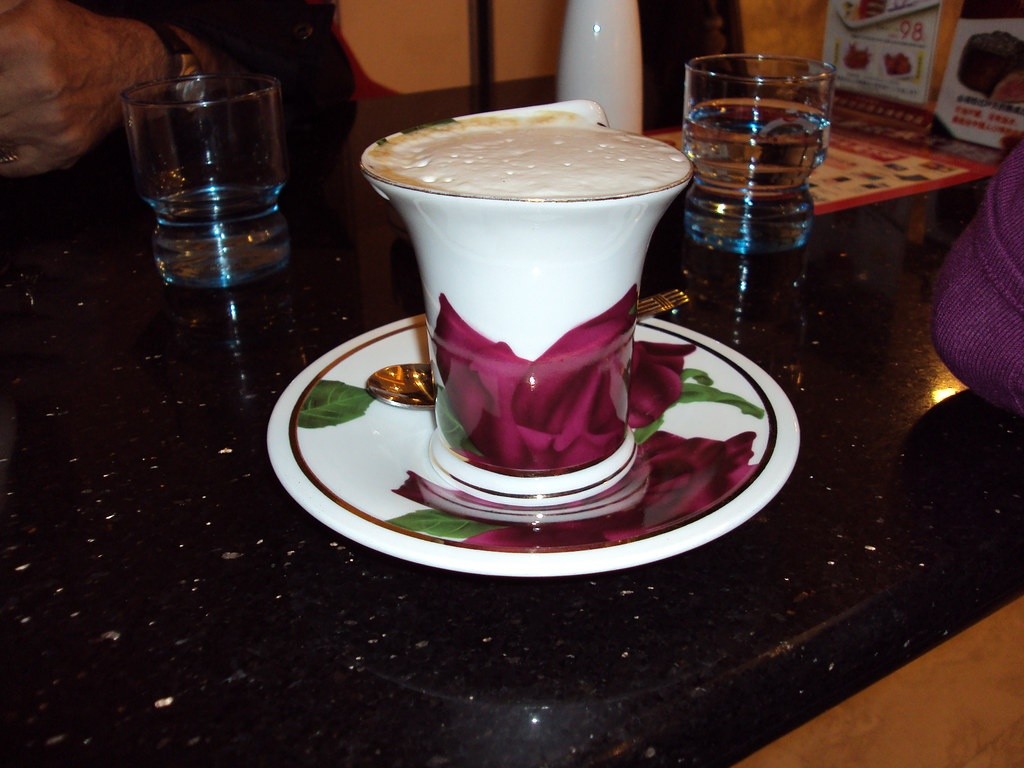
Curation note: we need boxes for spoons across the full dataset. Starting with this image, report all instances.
[365,288,690,409]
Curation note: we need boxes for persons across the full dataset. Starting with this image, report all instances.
[1,0,355,237]
[931,133,1024,419]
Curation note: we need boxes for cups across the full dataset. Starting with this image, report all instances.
[120,74,289,226]
[683,53,838,190]
[359,99,694,497]
[681,190,812,284]
[152,214,295,317]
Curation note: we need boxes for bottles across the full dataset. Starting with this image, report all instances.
[558,0,643,136]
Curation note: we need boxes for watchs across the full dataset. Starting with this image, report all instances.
[144,18,205,126]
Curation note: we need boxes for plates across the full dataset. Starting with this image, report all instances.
[267,311,802,577]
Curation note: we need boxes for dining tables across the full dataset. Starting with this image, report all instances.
[0,55,1024,768]
